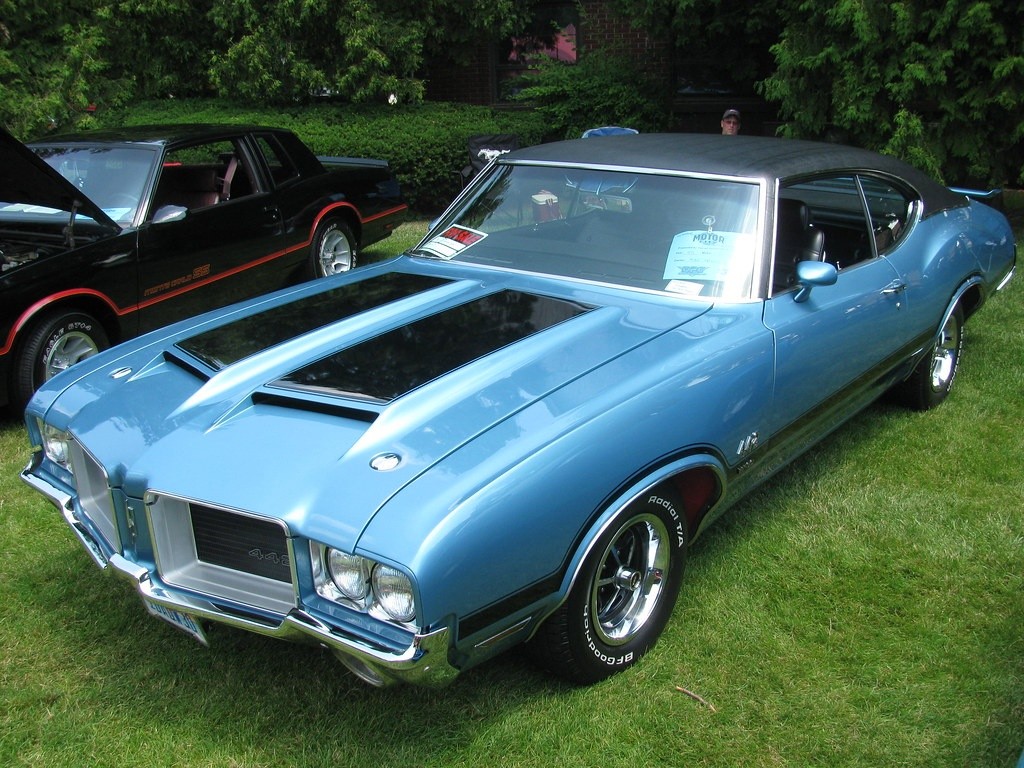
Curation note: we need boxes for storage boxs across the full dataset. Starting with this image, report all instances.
[531,189,558,223]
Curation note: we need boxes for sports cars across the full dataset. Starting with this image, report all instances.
[19,130,1020,694]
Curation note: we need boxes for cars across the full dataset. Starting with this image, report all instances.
[2,115,407,428]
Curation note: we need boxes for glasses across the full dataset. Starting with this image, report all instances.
[724,121,738,125]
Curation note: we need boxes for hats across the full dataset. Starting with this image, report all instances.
[722,108,741,124]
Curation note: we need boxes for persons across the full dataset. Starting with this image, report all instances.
[719,108,742,137]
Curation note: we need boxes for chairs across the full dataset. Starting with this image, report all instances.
[451,133,526,229]
[159,165,219,212]
[771,198,825,293]
[566,126,642,218]
